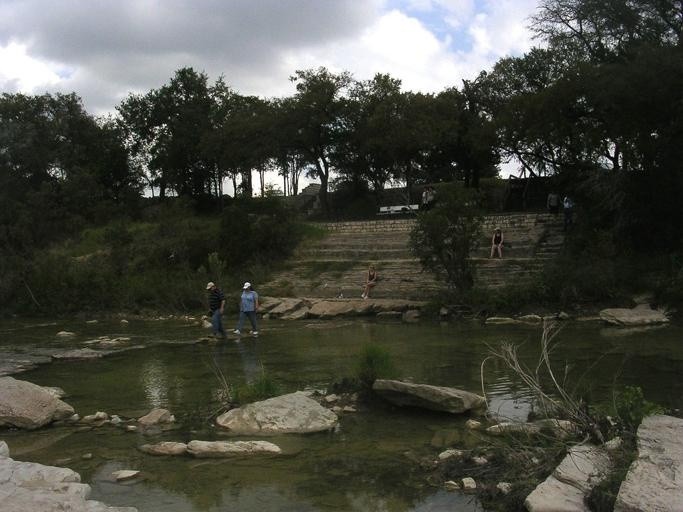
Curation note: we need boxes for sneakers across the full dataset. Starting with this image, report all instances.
[233,330,257,335]
[361,294,368,298]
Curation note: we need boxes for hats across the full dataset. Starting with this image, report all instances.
[243,282,251,289]
[205,282,214,289]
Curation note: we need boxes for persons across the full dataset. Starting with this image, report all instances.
[546,189,563,213]
[488,226,504,259]
[205,281,227,338]
[563,196,574,232]
[233,282,259,335]
[361,265,377,299]
[421,185,436,210]
